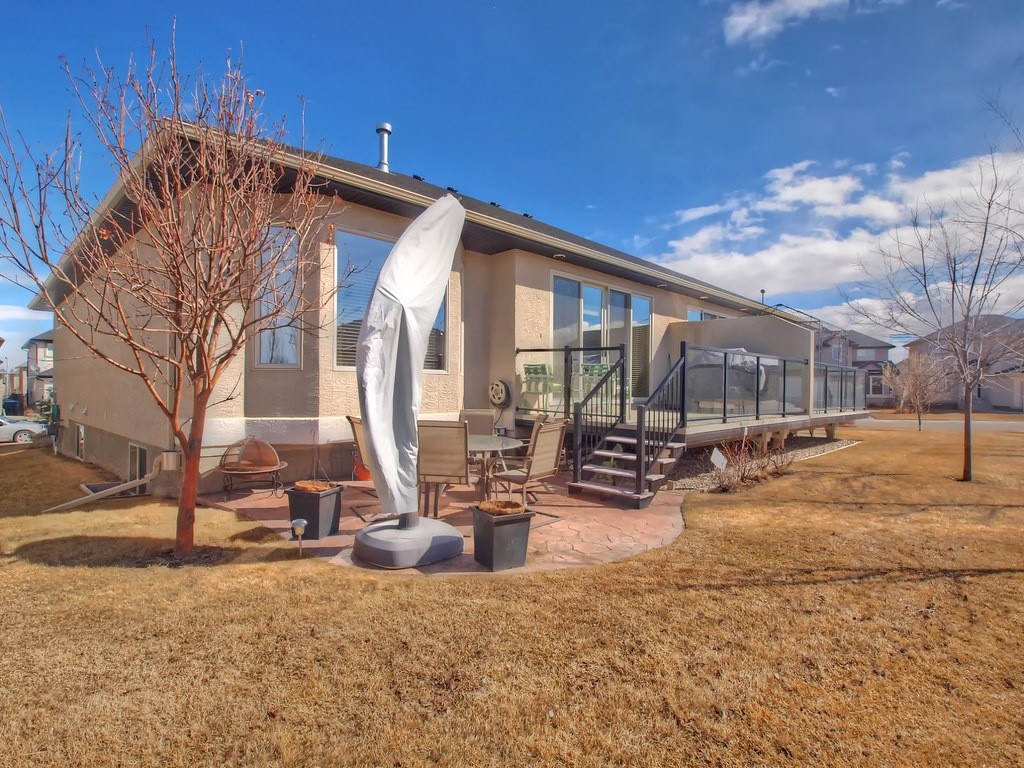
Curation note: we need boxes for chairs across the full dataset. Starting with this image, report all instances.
[521,363,631,420]
[345,409,572,520]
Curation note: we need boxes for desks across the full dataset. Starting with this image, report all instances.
[467,434,523,501]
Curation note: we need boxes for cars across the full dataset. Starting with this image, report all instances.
[0,416,48,444]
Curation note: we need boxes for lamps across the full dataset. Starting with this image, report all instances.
[291,519,308,556]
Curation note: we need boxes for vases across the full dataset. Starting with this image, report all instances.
[285,485,345,539]
[469,506,536,571]
